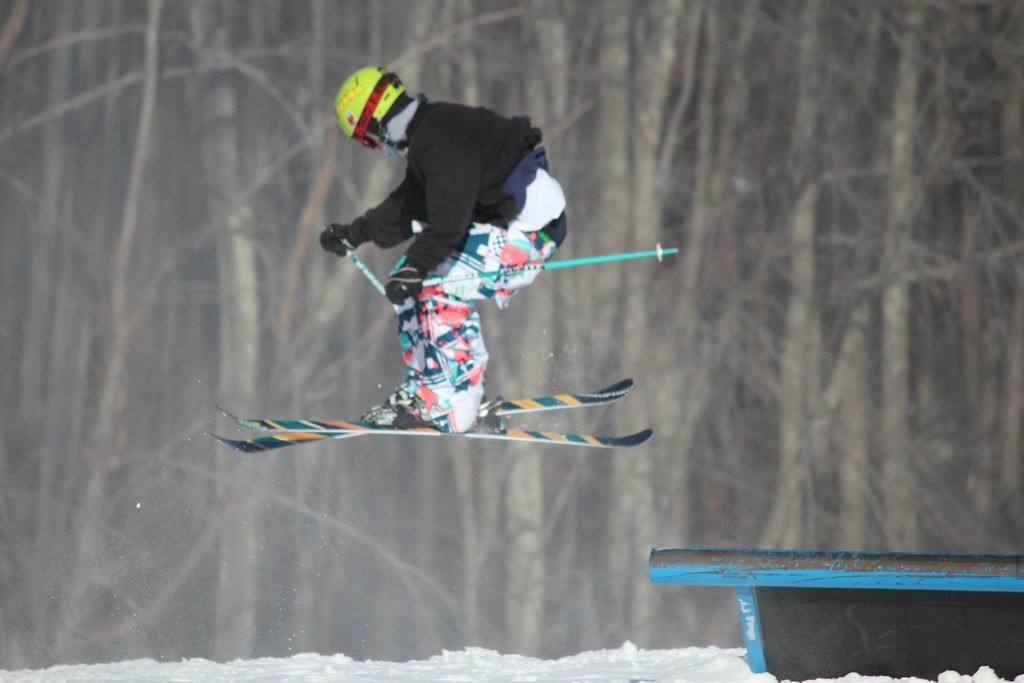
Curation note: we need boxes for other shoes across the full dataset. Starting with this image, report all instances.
[364,403,423,428]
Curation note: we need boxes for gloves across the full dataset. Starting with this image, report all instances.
[385,257,429,305]
[319,217,370,257]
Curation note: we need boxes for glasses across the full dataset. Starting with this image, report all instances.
[352,129,366,143]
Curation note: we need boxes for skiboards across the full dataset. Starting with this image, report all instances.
[205,378,656,454]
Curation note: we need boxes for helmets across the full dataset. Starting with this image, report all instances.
[333,66,405,150]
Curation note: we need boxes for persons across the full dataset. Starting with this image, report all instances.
[318,66,568,438]
